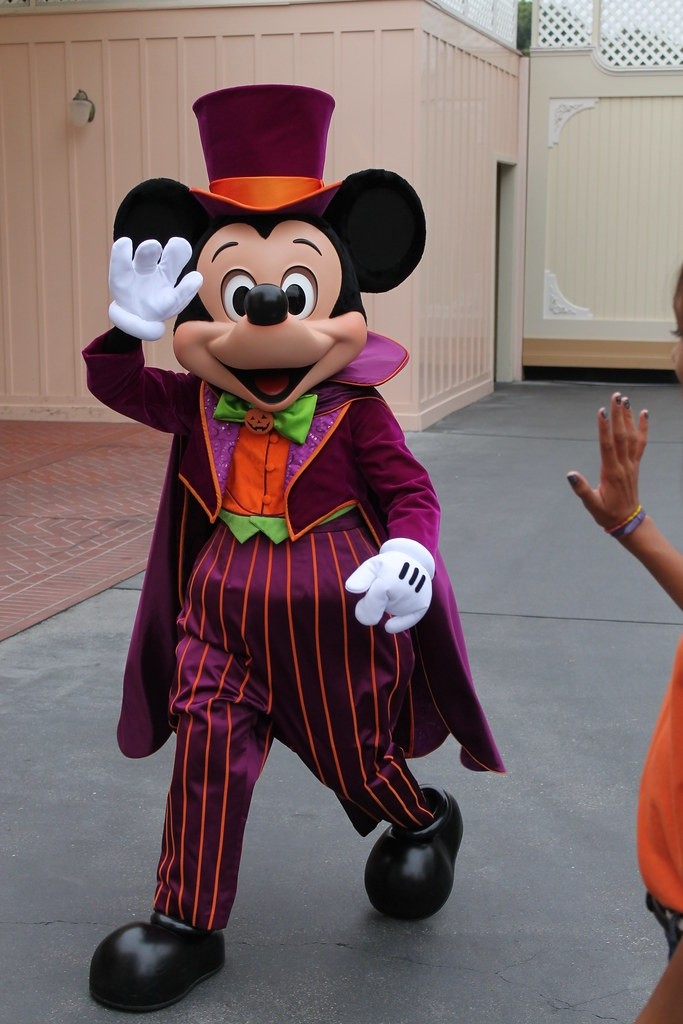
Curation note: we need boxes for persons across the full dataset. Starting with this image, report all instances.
[567,264,683,1024]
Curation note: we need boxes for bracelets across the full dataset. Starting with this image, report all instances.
[613,512,646,537]
[605,505,642,533]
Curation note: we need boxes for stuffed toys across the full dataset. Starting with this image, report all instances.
[81,84,507,1014]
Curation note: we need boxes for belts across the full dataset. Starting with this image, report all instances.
[646,893,683,931]
